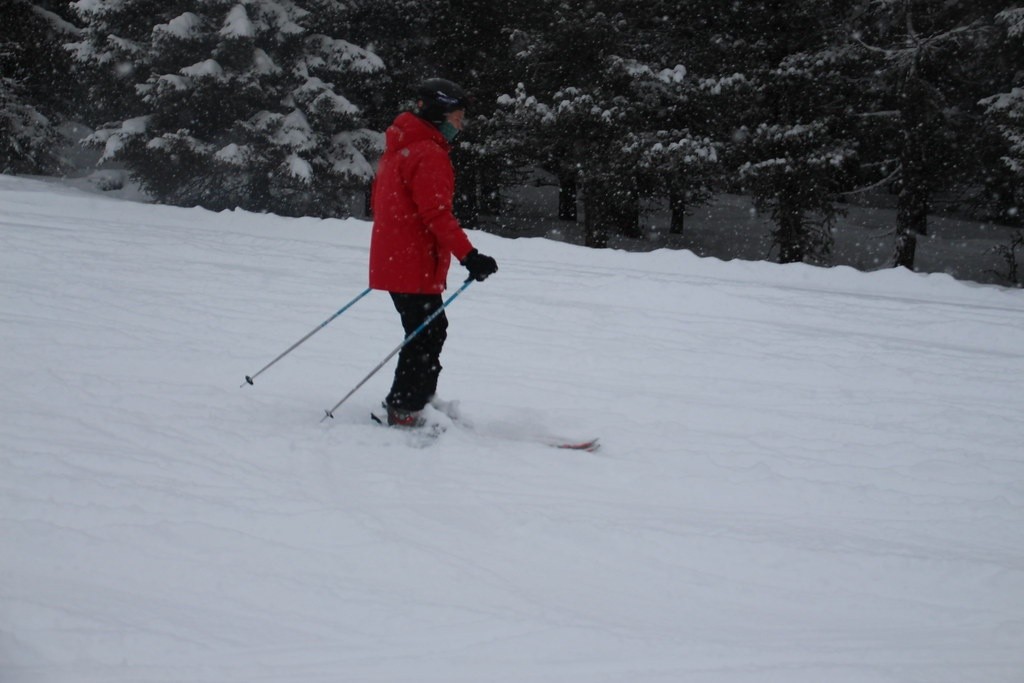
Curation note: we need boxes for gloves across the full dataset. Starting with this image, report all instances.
[461,249,498,281]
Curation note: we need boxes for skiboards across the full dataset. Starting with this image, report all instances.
[324,409,600,456]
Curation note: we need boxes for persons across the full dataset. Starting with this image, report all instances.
[369,78,499,428]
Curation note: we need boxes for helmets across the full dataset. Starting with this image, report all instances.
[416,78,476,124]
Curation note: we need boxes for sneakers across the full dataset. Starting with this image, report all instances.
[386,405,447,433]
